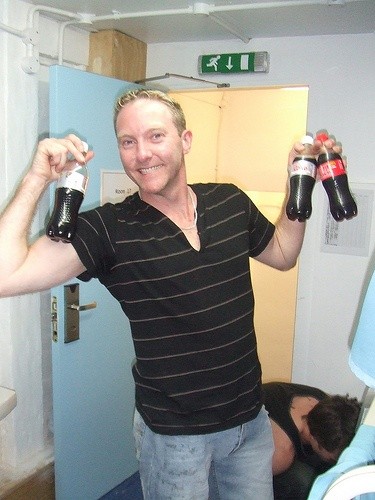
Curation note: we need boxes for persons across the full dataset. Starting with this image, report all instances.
[0,89,347,500]
[261,382,363,500]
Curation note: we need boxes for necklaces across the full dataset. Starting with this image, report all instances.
[137,187,198,230]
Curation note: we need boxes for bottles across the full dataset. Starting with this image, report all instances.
[46,140,89,243]
[287,136,316,223]
[315,133,357,222]
